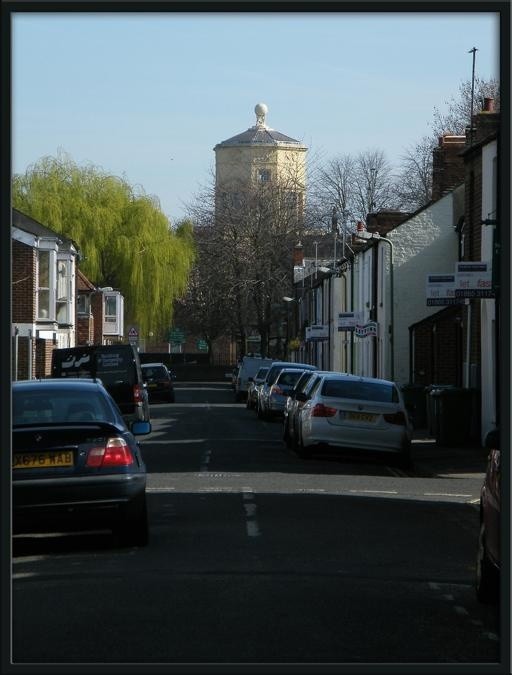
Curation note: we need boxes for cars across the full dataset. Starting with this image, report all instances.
[12,377,151,549]
[51,344,150,432]
[232,355,413,458]
[140,363,176,404]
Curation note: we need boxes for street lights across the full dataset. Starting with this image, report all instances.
[282,296,299,363]
[316,266,347,373]
[352,230,395,383]
